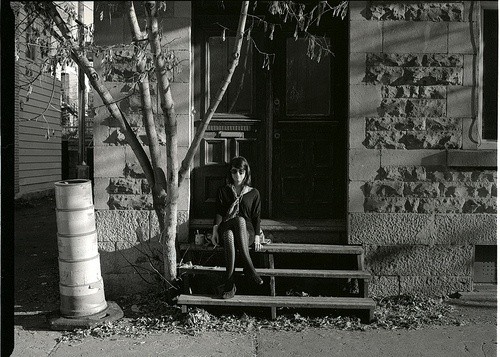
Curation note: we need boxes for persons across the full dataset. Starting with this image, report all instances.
[211,156,264,299]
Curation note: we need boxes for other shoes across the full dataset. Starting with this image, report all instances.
[222,284,237,298]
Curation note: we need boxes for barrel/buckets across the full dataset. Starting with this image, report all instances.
[53,180,109,319]
